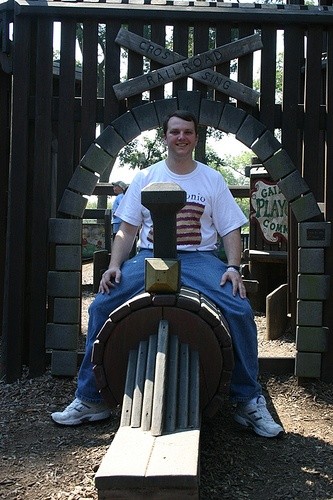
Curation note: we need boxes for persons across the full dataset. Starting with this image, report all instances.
[50,109,285,437]
[111,182,140,260]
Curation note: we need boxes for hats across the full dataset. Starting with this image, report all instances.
[112,180,128,191]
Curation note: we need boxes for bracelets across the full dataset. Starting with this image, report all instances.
[226,265,240,274]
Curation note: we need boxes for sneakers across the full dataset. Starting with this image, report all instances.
[234,393,285,437]
[51,398,112,425]
